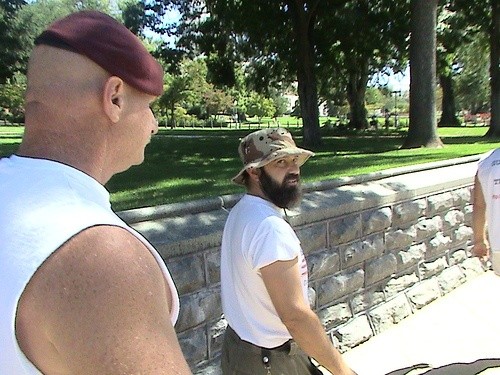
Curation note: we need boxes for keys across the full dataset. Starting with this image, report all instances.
[262,357,274,375]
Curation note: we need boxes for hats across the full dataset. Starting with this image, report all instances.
[32,9,164,97]
[230,128,316,186]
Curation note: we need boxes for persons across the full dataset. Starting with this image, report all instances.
[472,147,500,277]
[1,10,196,375]
[220,128,358,375]
[385,109,390,129]
[230,112,238,122]
